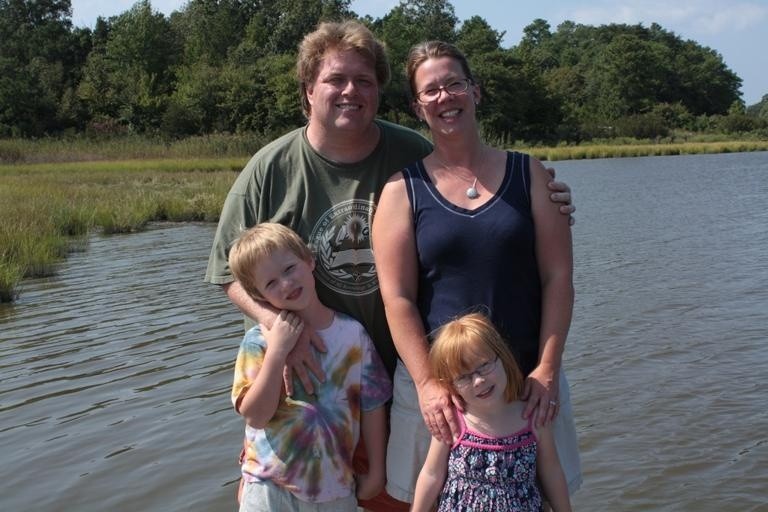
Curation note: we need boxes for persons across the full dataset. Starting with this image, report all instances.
[204,20,575,512]
[407,310,575,511]
[228,220,394,512]
[369,39,586,511]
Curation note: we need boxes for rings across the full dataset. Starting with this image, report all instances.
[548,401,557,406]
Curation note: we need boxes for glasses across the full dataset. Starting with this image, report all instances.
[415,77,472,104]
[452,352,501,388]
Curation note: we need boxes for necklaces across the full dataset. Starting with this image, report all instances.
[430,146,493,199]
